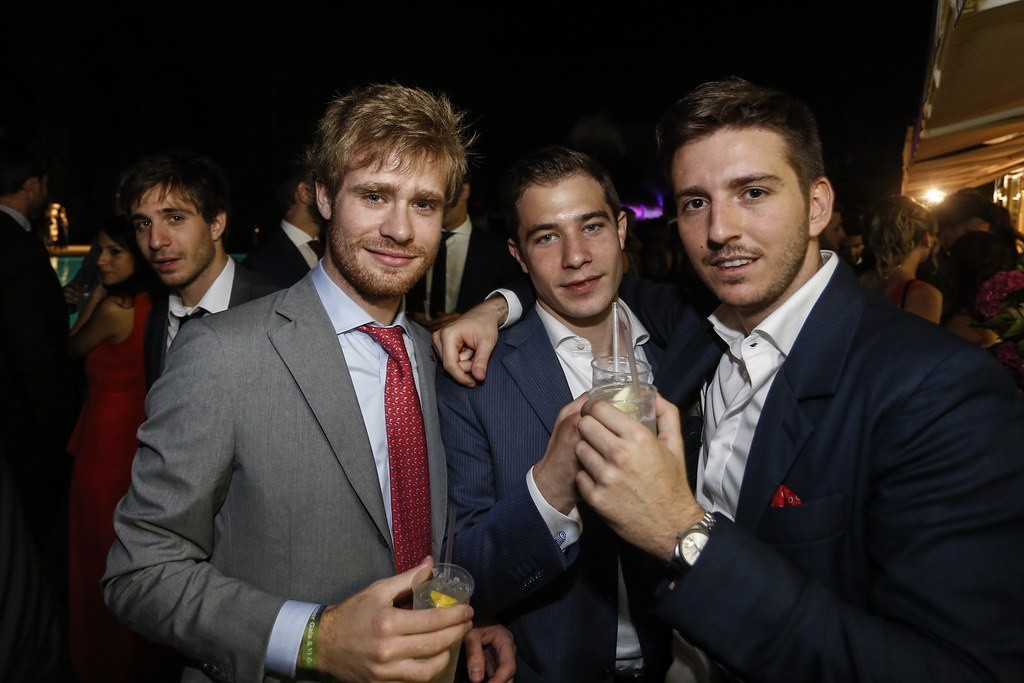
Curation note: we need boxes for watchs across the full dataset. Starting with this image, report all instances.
[670,510,715,573]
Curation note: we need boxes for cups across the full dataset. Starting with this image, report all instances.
[582,355,659,442]
[411,562,474,683]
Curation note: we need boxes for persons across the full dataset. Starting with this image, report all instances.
[98,85,517,683]
[435,147,665,683]
[119,153,278,399]
[241,142,327,289]
[819,187,1024,350]
[0,137,168,683]
[621,193,708,286]
[405,171,518,334]
[431,80,1024,683]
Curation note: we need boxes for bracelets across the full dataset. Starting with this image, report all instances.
[302,605,331,673]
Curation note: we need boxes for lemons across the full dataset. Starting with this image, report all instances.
[429,589,459,607]
[612,386,637,415]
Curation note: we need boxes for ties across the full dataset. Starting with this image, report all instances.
[178,308,206,330]
[428,232,455,319]
[307,240,323,262]
[354,323,431,609]
